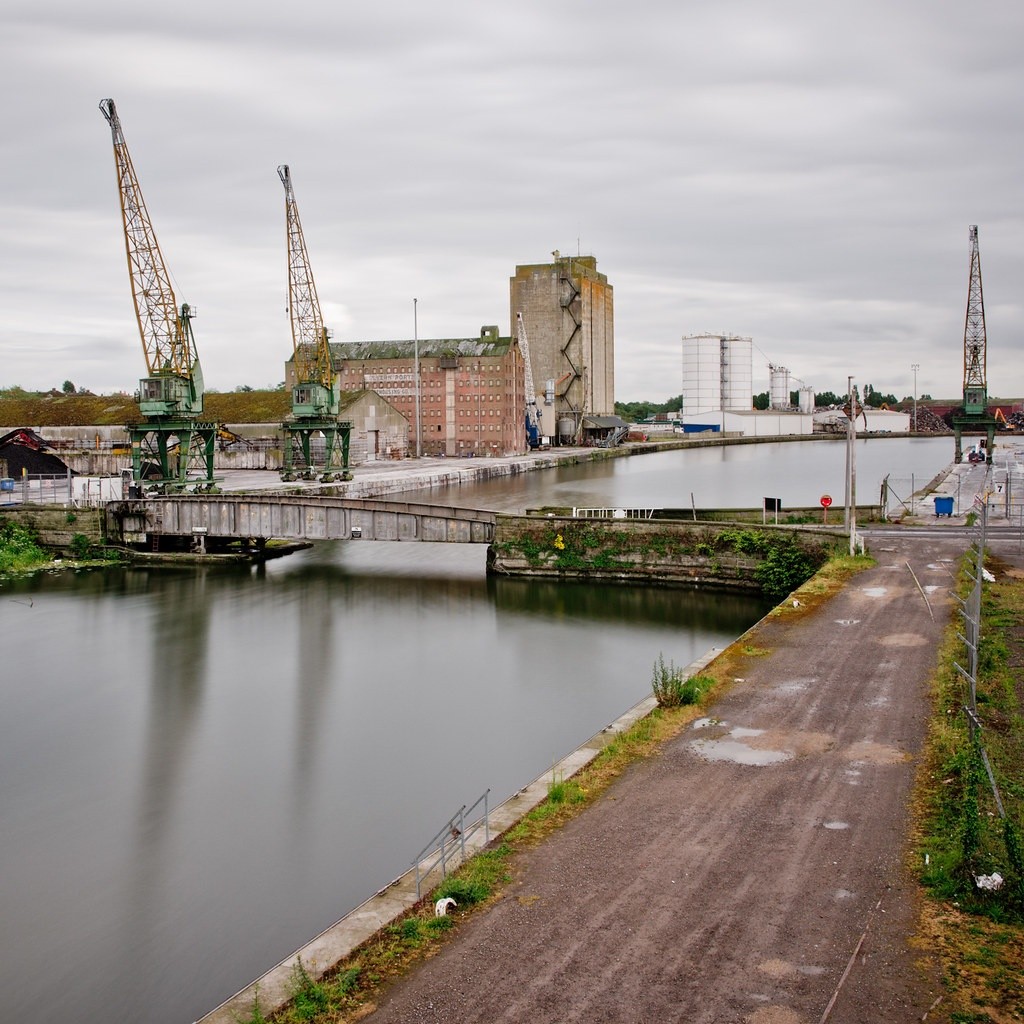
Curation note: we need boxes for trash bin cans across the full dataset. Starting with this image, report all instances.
[1,478,14,491]
[933,496,954,518]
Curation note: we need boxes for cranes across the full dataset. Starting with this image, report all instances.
[99,97,219,501]
[948,225,1005,466]
[276,164,355,483]
